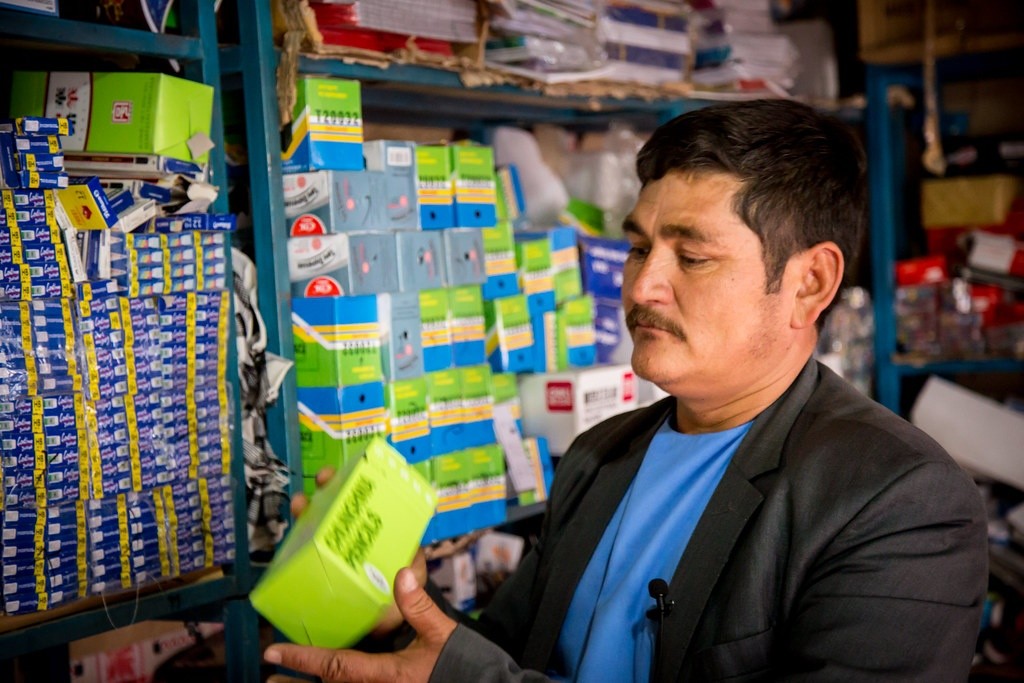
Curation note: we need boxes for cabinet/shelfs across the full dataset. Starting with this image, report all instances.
[0,0,257,683]
[238,0,900,683]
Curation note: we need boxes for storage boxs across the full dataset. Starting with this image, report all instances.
[3,71,669,650]
[922,181,1014,230]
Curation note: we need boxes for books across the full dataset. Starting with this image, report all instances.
[308,0,793,91]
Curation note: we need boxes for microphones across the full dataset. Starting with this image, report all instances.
[648,579,668,611]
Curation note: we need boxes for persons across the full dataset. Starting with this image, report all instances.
[263,97,990,683]
